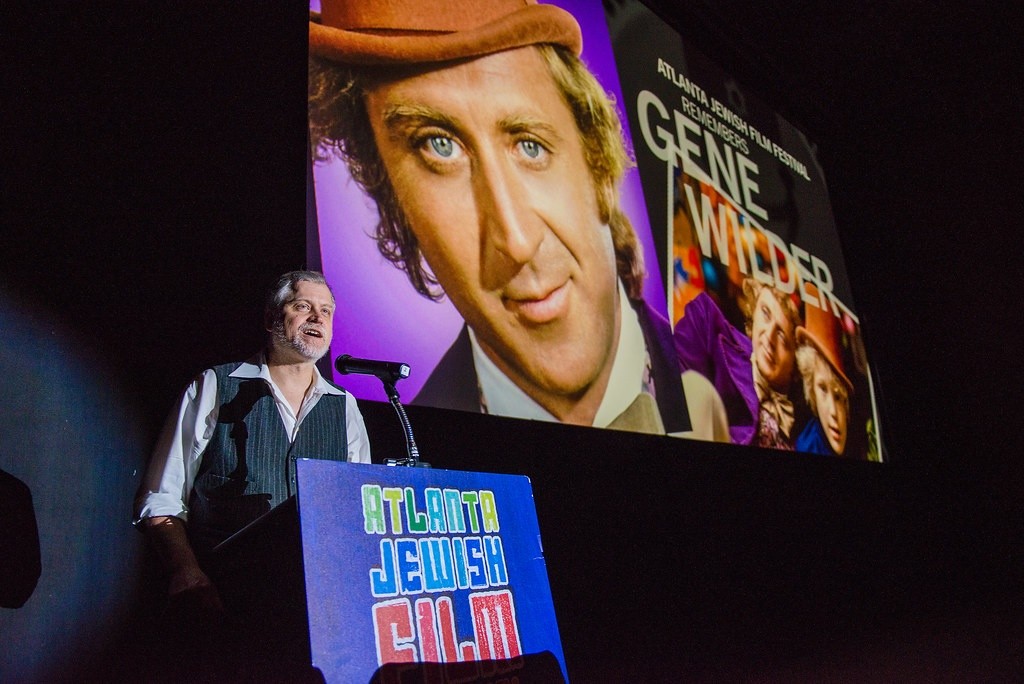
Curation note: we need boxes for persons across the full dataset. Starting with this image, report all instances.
[793,282,864,459]
[309,0,695,432]
[673,282,803,447]
[134,265,374,618]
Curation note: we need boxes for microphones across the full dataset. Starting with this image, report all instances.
[334,353,410,379]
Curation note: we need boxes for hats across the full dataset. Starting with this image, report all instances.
[795,282,854,393]
[309,0,583,65]
